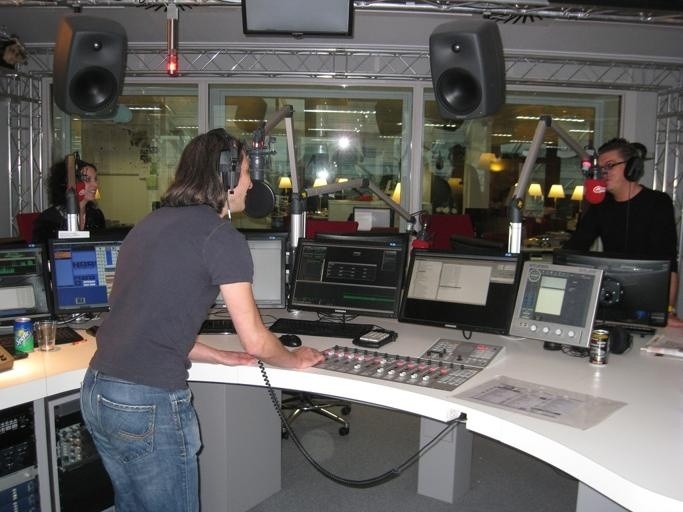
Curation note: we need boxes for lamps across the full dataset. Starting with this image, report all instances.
[527,183,583,221]
[278,175,349,211]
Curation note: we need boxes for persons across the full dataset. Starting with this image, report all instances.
[561,137,682,327]
[79,127,326,512]
[36,156,108,242]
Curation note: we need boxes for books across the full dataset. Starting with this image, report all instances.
[639,329,683,358]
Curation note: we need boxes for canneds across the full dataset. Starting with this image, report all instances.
[13,318,33,354]
[589,330,609,365]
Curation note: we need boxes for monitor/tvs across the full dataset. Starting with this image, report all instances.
[0,238,52,322]
[212,232,286,310]
[397,246,524,337]
[553,247,672,336]
[241,1,355,39]
[46,236,124,316]
[287,232,411,320]
[354,207,391,232]
[508,260,604,350]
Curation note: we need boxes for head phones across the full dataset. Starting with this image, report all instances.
[624,141,647,183]
[217,136,242,195]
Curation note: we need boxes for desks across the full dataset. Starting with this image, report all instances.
[0,308,683,512]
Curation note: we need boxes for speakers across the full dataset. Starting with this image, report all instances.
[234,97,267,132]
[375,104,401,135]
[52,14,128,120]
[429,19,506,120]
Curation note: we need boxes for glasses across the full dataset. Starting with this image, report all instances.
[600,159,628,169]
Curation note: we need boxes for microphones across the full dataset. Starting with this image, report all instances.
[241,128,276,219]
[583,165,607,205]
[74,167,86,202]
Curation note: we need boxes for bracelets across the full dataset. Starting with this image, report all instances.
[667,306,677,315]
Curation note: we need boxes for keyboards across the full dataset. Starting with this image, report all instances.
[197,318,236,337]
[0,326,84,353]
[268,317,378,338]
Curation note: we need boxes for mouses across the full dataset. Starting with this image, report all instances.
[86,325,99,337]
[278,334,302,348]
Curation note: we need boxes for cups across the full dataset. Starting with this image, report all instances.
[34,320,57,351]
